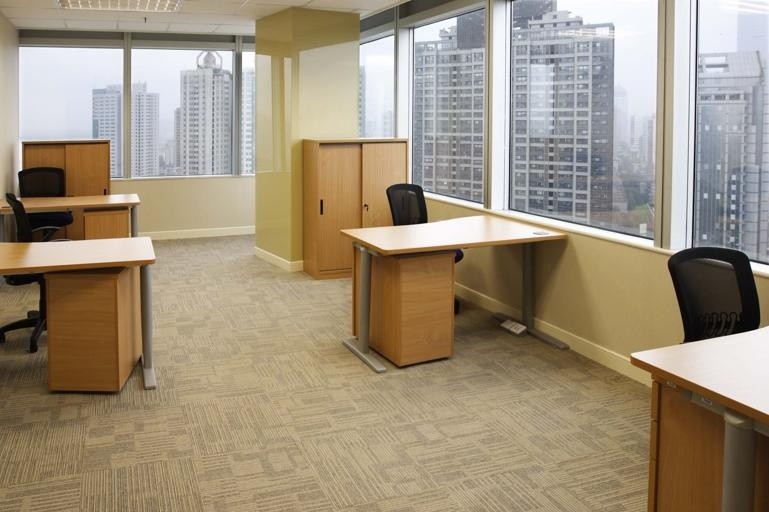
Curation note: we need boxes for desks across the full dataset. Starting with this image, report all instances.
[631,326,769,512]
[0,194,140,242]
[0,237,157,392]
[339,215,568,374]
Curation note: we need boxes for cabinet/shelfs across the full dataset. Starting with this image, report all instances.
[22,140,111,237]
[302,137,409,281]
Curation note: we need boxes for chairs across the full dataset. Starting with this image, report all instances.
[0,193,71,353]
[668,247,760,344]
[385,184,463,315]
[18,168,73,242]
